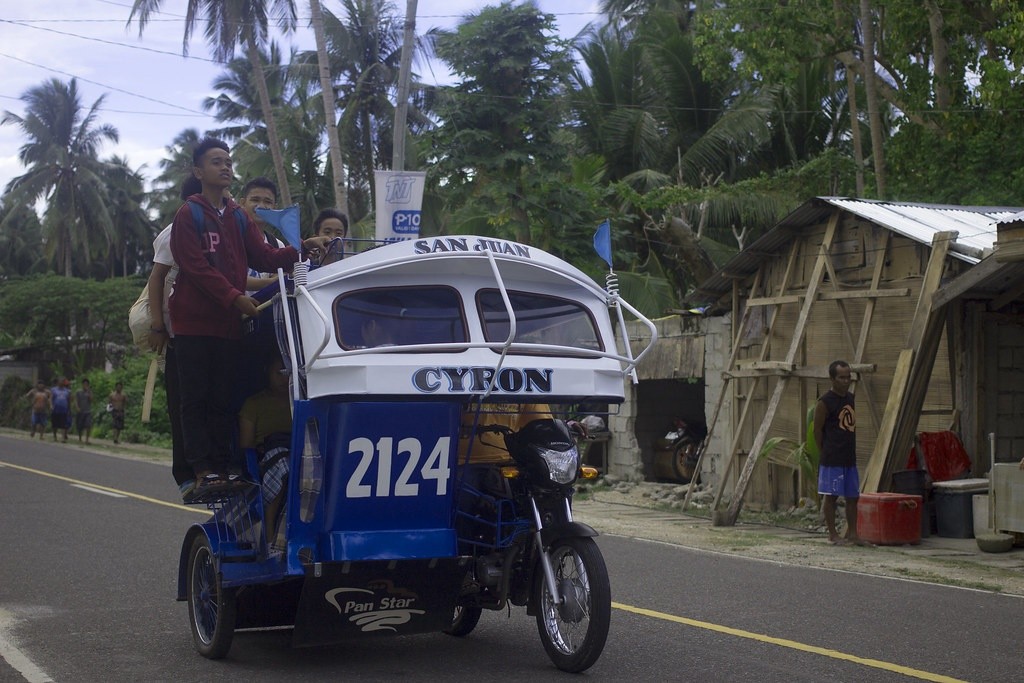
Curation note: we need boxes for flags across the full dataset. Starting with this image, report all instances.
[594,221,613,266]
[254,201,301,251]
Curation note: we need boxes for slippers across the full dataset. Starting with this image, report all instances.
[194,473,247,493]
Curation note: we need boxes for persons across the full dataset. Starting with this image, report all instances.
[813,360,876,550]
[107,382,128,444]
[50,378,73,440]
[26,380,51,440]
[149,138,590,608]
[74,379,93,444]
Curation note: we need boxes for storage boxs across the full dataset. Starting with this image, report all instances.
[932,477,990,538]
[855,492,922,545]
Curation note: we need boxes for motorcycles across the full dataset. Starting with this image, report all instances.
[664,415,708,485]
[176,217,656,672]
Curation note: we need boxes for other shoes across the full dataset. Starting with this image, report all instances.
[179,476,196,494]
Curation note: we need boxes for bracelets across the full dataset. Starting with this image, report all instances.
[151,326,166,334]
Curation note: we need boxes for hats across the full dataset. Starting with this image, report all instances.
[63,379,71,385]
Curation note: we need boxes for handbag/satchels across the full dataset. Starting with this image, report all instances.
[127,263,180,359]
